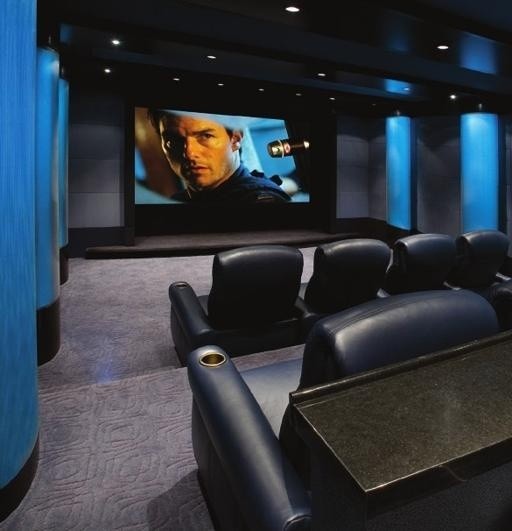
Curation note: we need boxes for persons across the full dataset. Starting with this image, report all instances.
[150,109,291,204]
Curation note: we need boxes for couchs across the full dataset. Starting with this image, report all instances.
[170,229,512,531]
